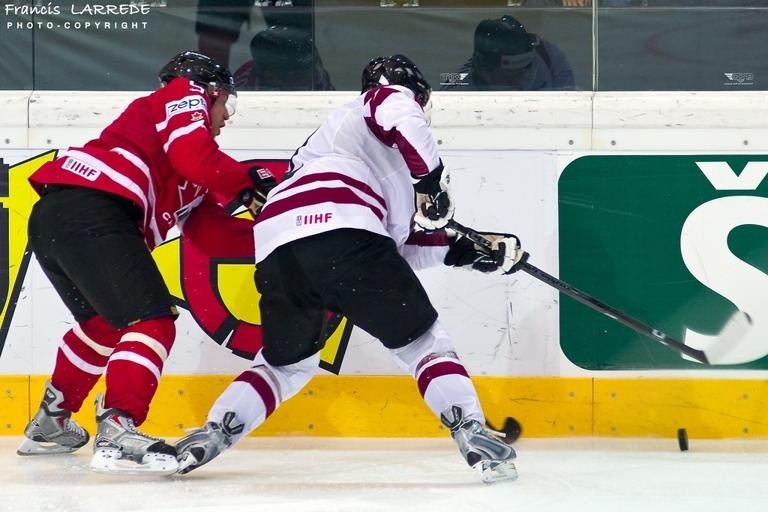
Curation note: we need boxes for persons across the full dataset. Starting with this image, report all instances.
[233,24,338,90]
[23,49,280,465]
[440,13,575,91]
[193,1,314,88]
[171,50,531,479]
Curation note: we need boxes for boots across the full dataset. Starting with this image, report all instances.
[23,381,90,448]
[173,412,244,474]
[93,395,176,458]
[441,406,517,467]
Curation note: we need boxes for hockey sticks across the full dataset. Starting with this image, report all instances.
[446,217,753,365]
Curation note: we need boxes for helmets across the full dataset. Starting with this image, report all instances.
[158,51,237,106]
[361,54,431,108]
[473,15,535,87]
[250,26,318,85]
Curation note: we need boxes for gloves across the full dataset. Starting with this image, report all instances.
[410,158,454,235]
[444,228,529,275]
